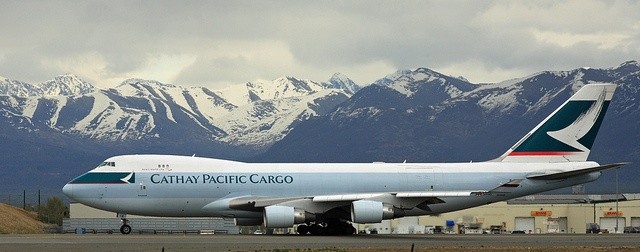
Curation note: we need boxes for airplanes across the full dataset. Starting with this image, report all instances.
[62,83,631,234]
